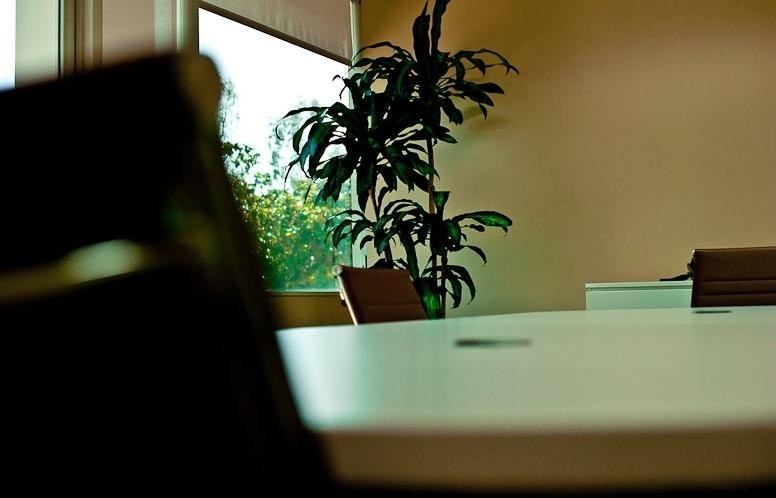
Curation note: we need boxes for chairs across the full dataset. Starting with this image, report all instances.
[332,264,428,326]
[0,51,345,498]
[687,247,776,307]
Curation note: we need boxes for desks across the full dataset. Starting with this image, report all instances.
[277,306,776,490]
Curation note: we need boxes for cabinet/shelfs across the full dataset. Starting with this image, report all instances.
[584,281,693,310]
